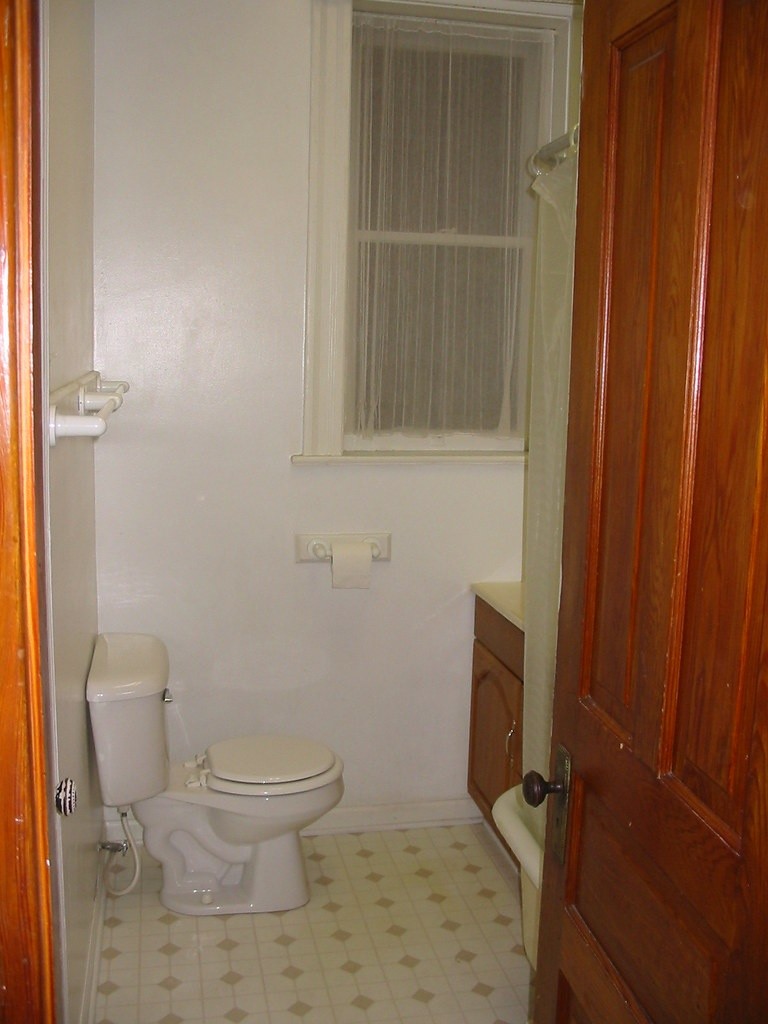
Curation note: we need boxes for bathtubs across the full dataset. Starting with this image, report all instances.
[490,776,547,970]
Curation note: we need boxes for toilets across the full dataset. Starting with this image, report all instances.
[84,631,345,916]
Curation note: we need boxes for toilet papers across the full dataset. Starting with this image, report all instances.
[330,545,375,591]
